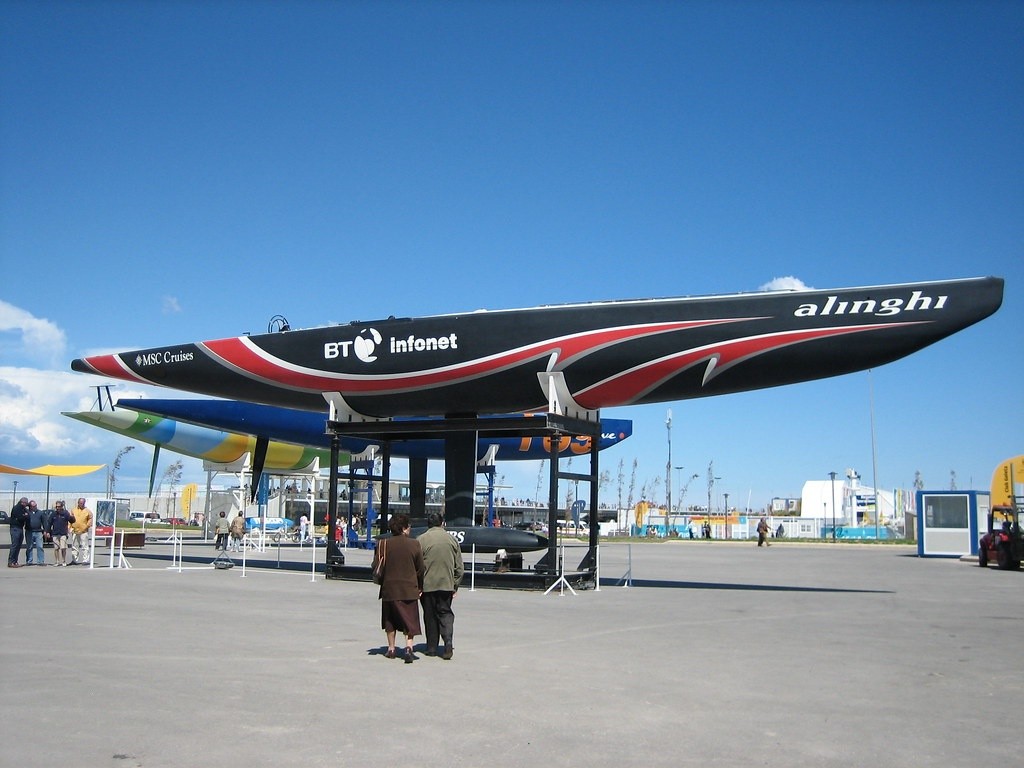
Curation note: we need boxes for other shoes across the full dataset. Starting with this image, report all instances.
[443,641,453,659]
[26,562,33,566]
[8,561,23,568]
[425,647,437,656]
[37,563,47,566]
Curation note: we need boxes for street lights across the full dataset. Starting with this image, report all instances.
[827,471,839,543]
[723,493,730,539]
[675,466,684,513]
[11,481,19,508]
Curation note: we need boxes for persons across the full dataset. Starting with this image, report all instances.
[214,510,246,553]
[401,484,445,504]
[415,512,464,659]
[599,502,617,510]
[481,513,584,537]
[323,512,361,544]
[243,478,325,504]
[668,519,712,539]
[493,495,532,506]
[298,514,311,543]
[646,525,659,538]
[371,512,425,663]
[8,497,93,568]
[756,516,784,548]
[339,489,348,501]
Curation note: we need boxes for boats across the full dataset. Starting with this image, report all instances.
[56,274,1005,476]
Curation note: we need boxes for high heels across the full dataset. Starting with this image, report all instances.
[405,646,413,663]
[385,649,396,658]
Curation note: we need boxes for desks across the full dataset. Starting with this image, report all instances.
[115,532,146,549]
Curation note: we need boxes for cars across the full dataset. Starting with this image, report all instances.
[161,518,186,525]
[129,511,161,524]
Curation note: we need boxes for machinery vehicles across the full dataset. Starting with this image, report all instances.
[977,463,1024,571]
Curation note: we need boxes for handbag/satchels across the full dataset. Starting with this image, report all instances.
[372,538,387,584]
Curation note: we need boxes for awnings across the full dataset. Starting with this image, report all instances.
[0,462,110,477]
